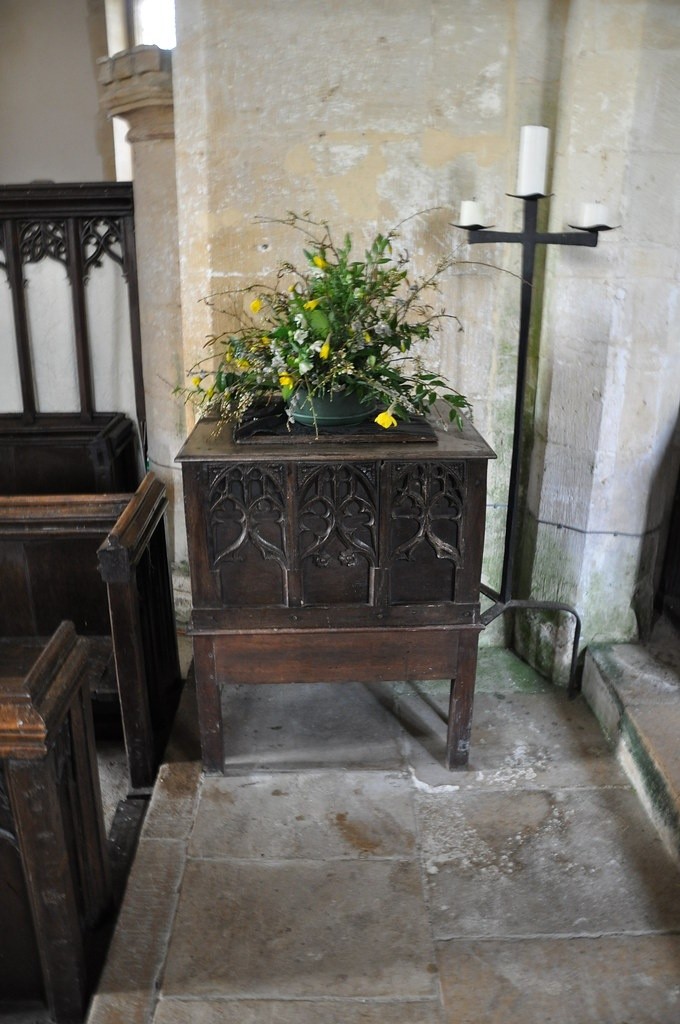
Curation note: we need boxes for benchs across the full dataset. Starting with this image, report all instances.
[0,473,182,795]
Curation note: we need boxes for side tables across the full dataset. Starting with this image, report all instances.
[174,402,499,775]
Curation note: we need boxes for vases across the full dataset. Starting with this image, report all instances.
[288,382,377,427]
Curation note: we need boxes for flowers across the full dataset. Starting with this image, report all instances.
[168,206,536,441]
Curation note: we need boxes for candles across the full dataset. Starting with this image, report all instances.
[582,200,605,228]
[457,195,484,227]
[515,124,550,197]
